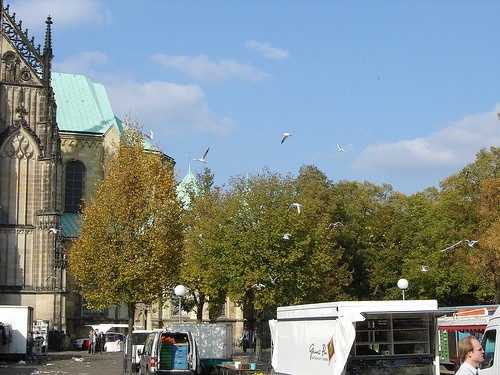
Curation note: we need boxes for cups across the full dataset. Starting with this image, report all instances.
[235,361,241,369]
[251,362,257,370]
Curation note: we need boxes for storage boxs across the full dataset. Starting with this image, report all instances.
[159,339,188,370]
[239,364,249,369]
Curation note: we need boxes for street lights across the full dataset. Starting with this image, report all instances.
[397,278,409,301]
[174,285,186,324]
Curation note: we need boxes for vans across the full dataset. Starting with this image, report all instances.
[125,328,202,375]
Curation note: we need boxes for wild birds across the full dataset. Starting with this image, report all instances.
[438,239,480,253]
[269,274,284,284]
[282,233,292,240]
[327,221,345,230]
[280,132,294,145]
[250,283,267,291]
[286,202,303,215]
[238,329,256,343]
[191,146,211,165]
[336,143,346,153]
[47,226,62,235]
[143,127,155,142]
[420,264,429,272]
[46,275,59,282]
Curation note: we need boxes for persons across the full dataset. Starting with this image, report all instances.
[242,326,250,352]
[48,330,72,351]
[253,327,257,352]
[88,329,105,355]
[26,331,34,363]
[455,335,485,375]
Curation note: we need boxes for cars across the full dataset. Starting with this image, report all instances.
[74,332,126,353]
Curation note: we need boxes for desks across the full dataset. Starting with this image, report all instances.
[215,364,262,375]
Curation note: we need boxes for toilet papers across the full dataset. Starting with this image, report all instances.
[235,362,241,368]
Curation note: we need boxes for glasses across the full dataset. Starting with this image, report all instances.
[467,347,484,355]
